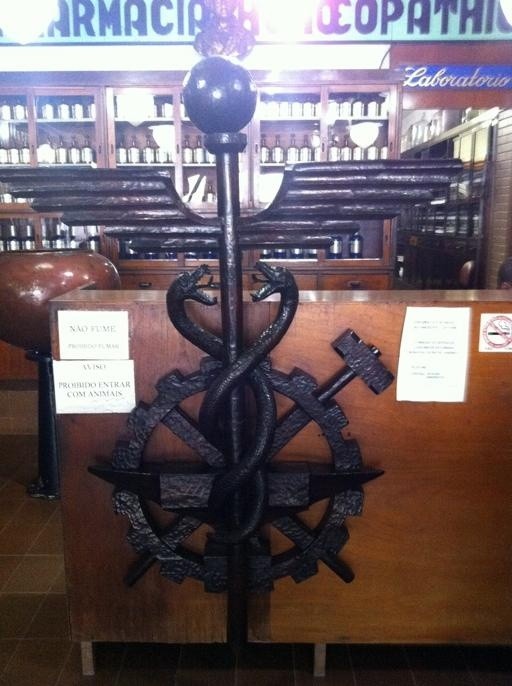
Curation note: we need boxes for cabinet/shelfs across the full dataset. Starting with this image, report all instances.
[397,106,499,242]
[0,79,403,271]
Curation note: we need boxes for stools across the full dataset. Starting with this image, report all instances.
[1,248,124,500]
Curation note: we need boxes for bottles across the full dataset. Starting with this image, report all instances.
[0,218,102,254]
[0,96,97,164]
[116,233,364,259]
[259,91,389,163]
[153,93,186,119]
[115,133,216,164]
[0,183,35,205]
